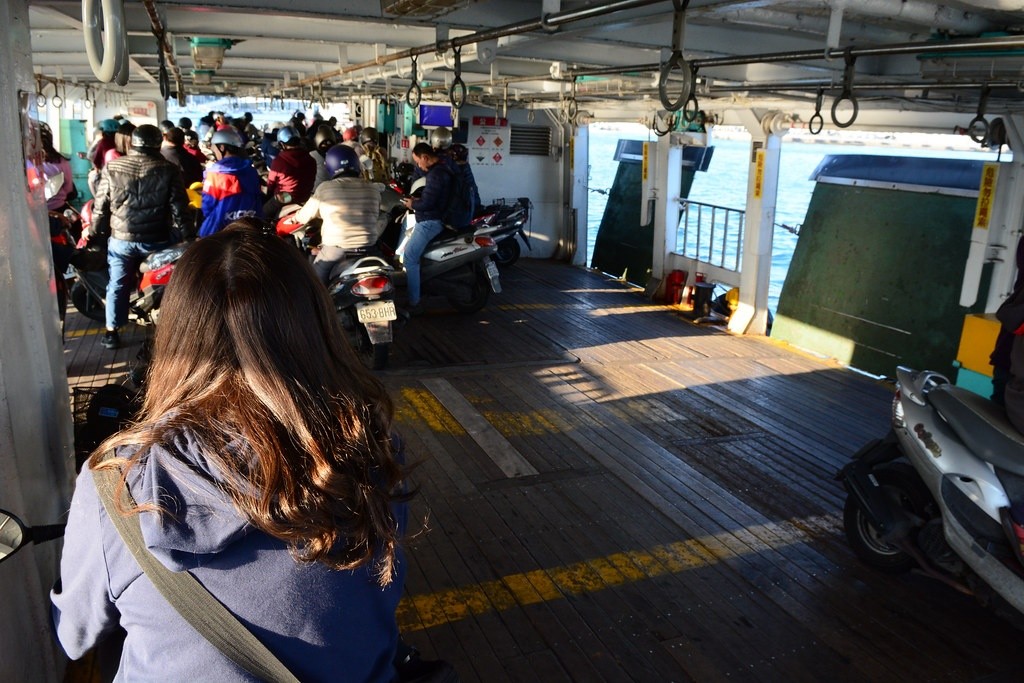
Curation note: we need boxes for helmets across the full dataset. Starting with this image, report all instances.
[359,126,380,145]
[431,126,452,149]
[165,126,185,145]
[313,128,337,148]
[213,112,223,120]
[218,124,233,130]
[99,119,121,133]
[178,117,192,129]
[119,118,133,125]
[277,125,302,143]
[342,126,358,141]
[132,124,163,147]
[324,143,361,180]
[270,122,286,134]
[159,119,175,134]
[211,129,244,148]
[185,130,199,146]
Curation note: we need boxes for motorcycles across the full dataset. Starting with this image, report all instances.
[0,383,461,683]
[42,201,187,330]
[277,191,536,370]
[833,365,1024,630]
[186,179,276,234]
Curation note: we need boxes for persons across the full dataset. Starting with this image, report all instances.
[39,121,72,332]
[83,109,264,349]
[268,110,481,222]
[404,142,457,313]
[291,146,380,283]
[48,216,410,683]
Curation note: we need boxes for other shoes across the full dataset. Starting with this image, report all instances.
[403,301,424,316]
[100,333,117,349]
